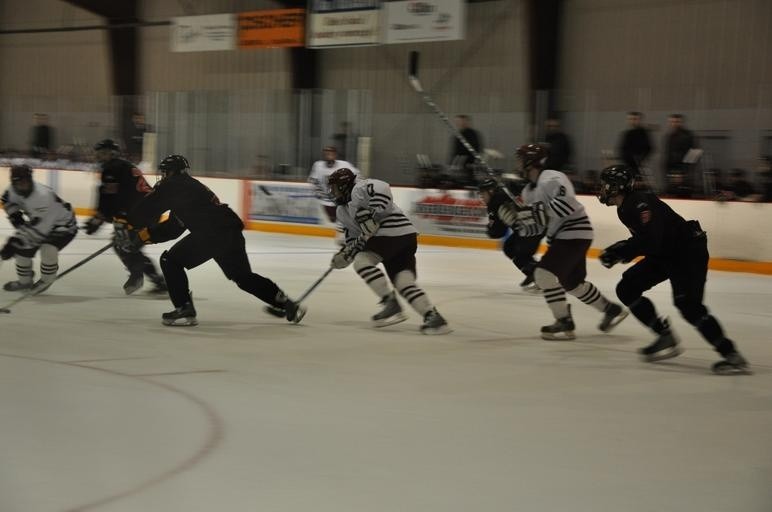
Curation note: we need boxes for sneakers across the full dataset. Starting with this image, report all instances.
[372,290,402,320]
[420,306,447,329]
[147,277,173,295]
[520,271,541,286]
[3,279,33,291]
[712,354,747,370]
[600,302,622,333]
[286,298,299,321]
[122,270,143,289]
[162,302,195,320]
[641,331,680,354]
[29,277,55,295]
[540,315,575,333]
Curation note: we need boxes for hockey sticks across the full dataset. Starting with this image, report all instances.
[406,50,524,212]
[262,264,333,317]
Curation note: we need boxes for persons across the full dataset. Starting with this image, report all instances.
[325,167,451,327]
[492,143,623,331]
[415,163,457,190]
[543,118,579,190]
[653,111,704,195]
[472,178,547,289]
[614,111,656,187]
[593,165,747,369]
[81,139,166,294]
[111,152,300,322]
[561,155,771,203]
[304,145,363,244]
[1,164,79,297]
[444,114,488,187]
[329,120,361,164]
[27,112,58,159]
[126,112,159,166]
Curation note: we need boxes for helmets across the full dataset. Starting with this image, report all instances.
[479,178,502,203]
[323,145,336,160]
[597,164,634,203]
[157,154,189,182]
[94,138,119,163]
[327,168,355,204]
[515,141,550,177]
[10,164,33,198]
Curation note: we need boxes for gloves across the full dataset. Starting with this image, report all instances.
[0,237,23,261]
[6,202,24,228]
[499,201,544,237]
[599,239,631,270]
[83,213,104,235]
[333,245,357,270]
[114,227,151,255]
